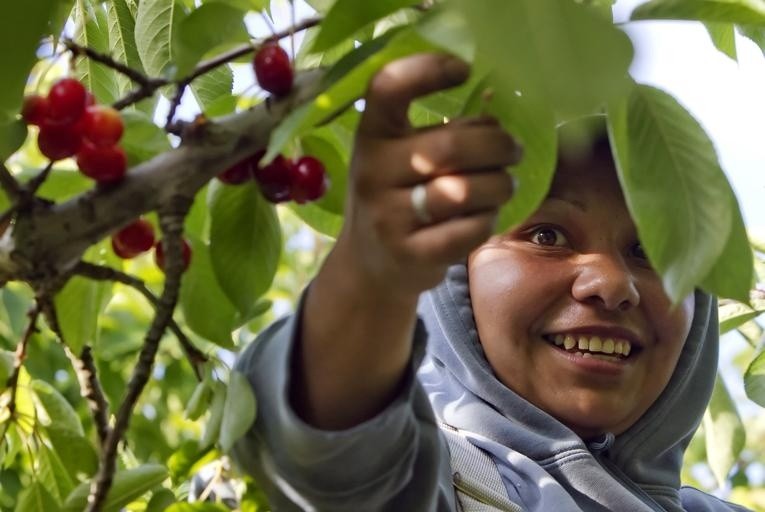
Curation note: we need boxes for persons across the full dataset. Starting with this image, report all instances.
[230,52,755,512]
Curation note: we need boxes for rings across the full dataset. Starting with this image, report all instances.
[410,183,433,227]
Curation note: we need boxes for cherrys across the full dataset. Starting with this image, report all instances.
[254,148,294,200]
[21,48,125,179]
[294,155,328,204]
[253,35,293,95]
[112,220,155,259]
[154,235,193,274]
[217,159,254,183]
